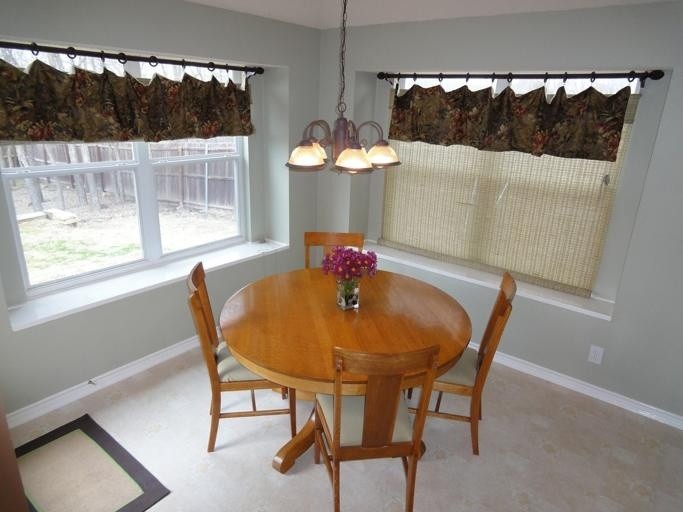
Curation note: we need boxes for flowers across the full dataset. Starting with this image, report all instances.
[320,244,377,298]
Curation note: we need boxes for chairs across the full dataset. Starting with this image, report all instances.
[186,259,297,451]
[316,343,440,512]
[402,270,517,456]
[304,231,365,273]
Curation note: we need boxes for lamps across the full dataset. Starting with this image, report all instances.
[284,1,401,174]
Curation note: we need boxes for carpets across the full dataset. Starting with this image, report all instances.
[15,415,171,512]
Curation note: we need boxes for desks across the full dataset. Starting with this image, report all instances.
[219,267,474,477]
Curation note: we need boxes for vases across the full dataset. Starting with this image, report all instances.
[336,279,360,311]
